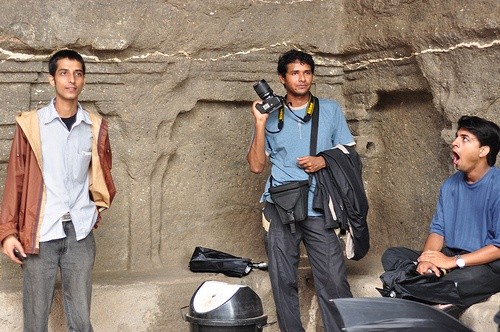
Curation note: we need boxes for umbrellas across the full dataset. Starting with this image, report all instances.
[186,246,269,279]
[375,260,462,304]
[328,297,473,332]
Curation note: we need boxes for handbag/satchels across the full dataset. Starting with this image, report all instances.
[269,180,310,234]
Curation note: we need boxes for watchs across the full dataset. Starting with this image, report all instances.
[455,253,466,270]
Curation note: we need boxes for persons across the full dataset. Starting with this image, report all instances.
[381,115,500,305]
[247,49,355,332]
[0,50,118,331]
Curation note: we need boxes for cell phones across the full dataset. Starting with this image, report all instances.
[14,249,25,262]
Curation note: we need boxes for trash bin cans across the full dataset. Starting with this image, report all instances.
[180,280,268,332]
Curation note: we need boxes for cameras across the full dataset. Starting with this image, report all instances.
[253,79,283,114]
